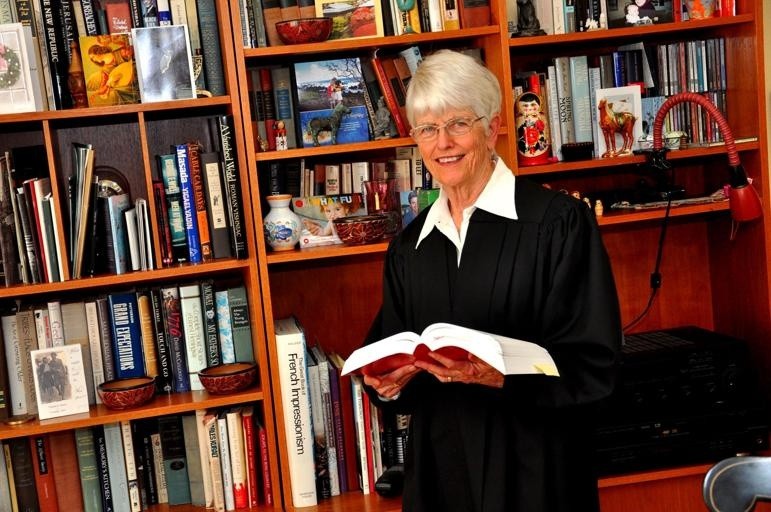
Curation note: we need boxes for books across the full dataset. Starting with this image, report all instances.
[274,314,416,510]
[242,1,491,249]
[339,321,561,383]
[275,318,412,508]
[1,0,227,118]
[1,115,274,512]
[506,1,761,166]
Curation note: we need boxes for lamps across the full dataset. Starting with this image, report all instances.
[621,92,765,333]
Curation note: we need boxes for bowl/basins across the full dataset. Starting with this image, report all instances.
[275,18,335,46]
[332,214,389,247]
[94,375,159,411]
[197,360,262,397]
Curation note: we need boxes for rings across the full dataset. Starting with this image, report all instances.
[446,374,453,383]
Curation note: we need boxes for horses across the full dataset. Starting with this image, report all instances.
[307,103,352,147]
[598,98,636,158]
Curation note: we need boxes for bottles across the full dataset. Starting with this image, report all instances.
[364,175,402,236]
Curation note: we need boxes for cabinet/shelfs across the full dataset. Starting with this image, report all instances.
[226,0,510,511]
[0,0,282,511]
[498,0,771,512]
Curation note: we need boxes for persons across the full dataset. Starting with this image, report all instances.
[359,47,625,510]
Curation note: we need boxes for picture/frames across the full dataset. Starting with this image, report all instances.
[0,22,36,114]
[594,84,643,160]
[313,0,384,42]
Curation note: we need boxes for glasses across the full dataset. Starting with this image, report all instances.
[409,116,490,143]
